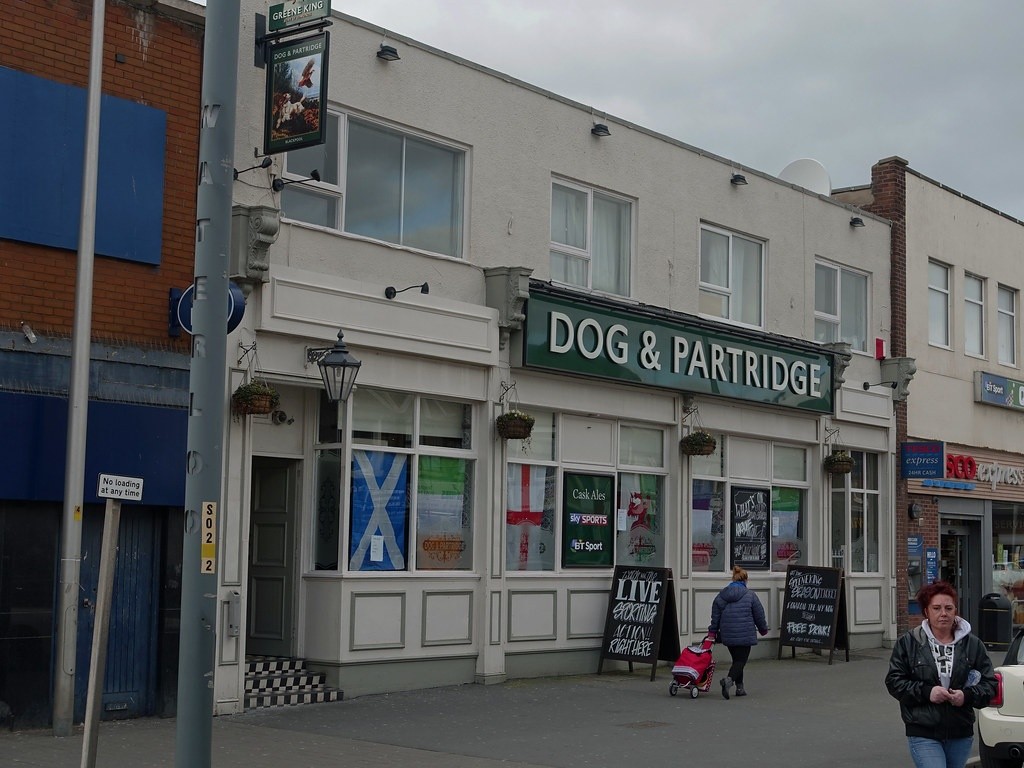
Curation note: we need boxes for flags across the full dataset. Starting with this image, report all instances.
[506,463,555,570]
[693,478,715,571]
[417,455,473,568]
[772,486,800,572]
[617,473,657,565]
[350,450,406,570]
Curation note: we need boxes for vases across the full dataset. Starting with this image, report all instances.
[827,462,851,473]
[497,420,534,439]
[681,442,715,455]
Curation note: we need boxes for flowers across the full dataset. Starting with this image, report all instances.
[496,410,536,457]
[679,431,719,460]
[823,452,857,479]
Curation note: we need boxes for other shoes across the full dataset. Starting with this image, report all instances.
[720,677,734,700]
[735,685,747,696]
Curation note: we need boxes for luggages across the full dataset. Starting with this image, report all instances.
[668,636,717,698]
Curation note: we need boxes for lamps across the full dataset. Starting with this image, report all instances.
[304,328,362,403]
[591,124,611,136]
[233,157,272,181]
[863,381,898,390]
[273,170,320,192]
[731,175,748,185]
[377,45,400,61]
[385,282,429,300]
[850,218,865,227]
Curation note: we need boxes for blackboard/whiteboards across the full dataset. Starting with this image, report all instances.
[778,564,850,651]
[599,565,681,663]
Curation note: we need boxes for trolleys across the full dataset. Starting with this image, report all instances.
[669,635,717,698]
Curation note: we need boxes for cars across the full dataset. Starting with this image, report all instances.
[975,627,1024,768]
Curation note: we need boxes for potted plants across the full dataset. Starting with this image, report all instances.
[231,380,281,426]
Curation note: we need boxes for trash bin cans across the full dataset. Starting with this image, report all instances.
[978,592,1013,652]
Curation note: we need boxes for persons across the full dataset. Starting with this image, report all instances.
[709,567,767,699]
[884,581,999,768]
